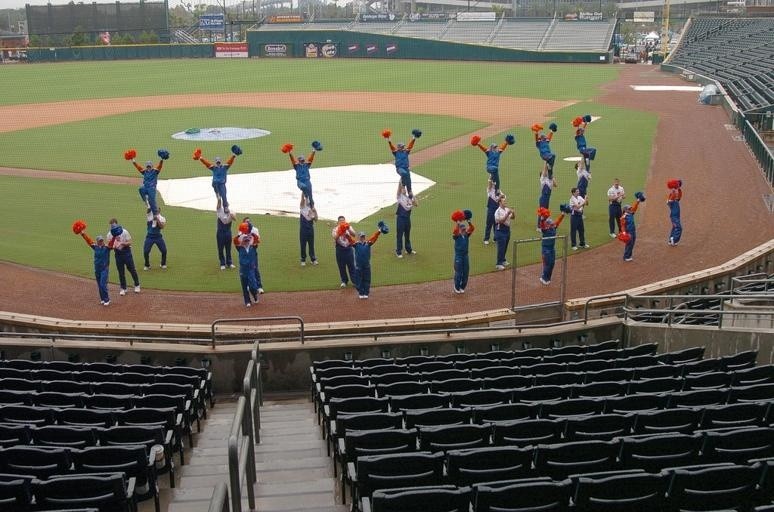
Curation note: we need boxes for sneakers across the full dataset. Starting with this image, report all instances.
[224,207,229,213]
[459,286,464,293]
[226,263,235,269]
[502,261,510,267]
[609,233,616,238]
[306,198,310,204]
[624,257,632,262]
[307,196,310,205]
[405,250,416,254]
[160,264,167,268]
[484,241,489,245]
[536,228,542,232]
[671,237,675,247]
[143,266,151,270]
[120,287,128,296]
[407,196,412,205]
[259,288,264,294]
[220,266,225,270]
[246,303,251,308]
[216,194,222,199]
[152,221,157,228]
[496,265,505,270]
[101,300,104,304]
[104,300,111,306]
[585,157,590,167]
[310,261,319,265]
[145,200,150,208]
[341,283,346,288]
[572,246,577,250]
[301,262,306,266]
[668,242,677,246]
[359,295,363,298]
[396,252,403,258]
[309,210,315,219]
[254,298,259,304]
[579,244,589,248]
[539,278,550,285]
[134,286,140,293]
[400,186,405,192]
[363,294,368,299]
[454,286,460,294]
[547,163,552,169]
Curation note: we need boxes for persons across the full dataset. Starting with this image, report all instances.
[624,40,664,66]
[343,221,390,299]
[73,221,123,306]
[106,219,141,296]
[299,191,320,266]
[665,179,683,246]
[142,194,168,271]
[192,145,265,308]
[281,140,324,223]
[451,114,645,294]
[382,128,423,207]
[125,149,169,228]
[395,177,417,258]
[331,216,357,288]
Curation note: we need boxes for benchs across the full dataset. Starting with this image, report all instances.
[666,14,774,114]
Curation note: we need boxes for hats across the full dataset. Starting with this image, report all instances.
[397,143,405,148]
[579,128,584,132]
[298,155,305,161]
[215,157,222,164]
[624,205,632,210]
[359,232,365,236]
[541,134,546,138]
[547,218,553,225]
[459,224,466,230]
[145,161,153,169]
[96,236,104,242]
[491,143,498,148]
[243,236,250,242]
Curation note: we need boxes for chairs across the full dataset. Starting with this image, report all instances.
[0,359,215,512]
[630,273,774,325]
